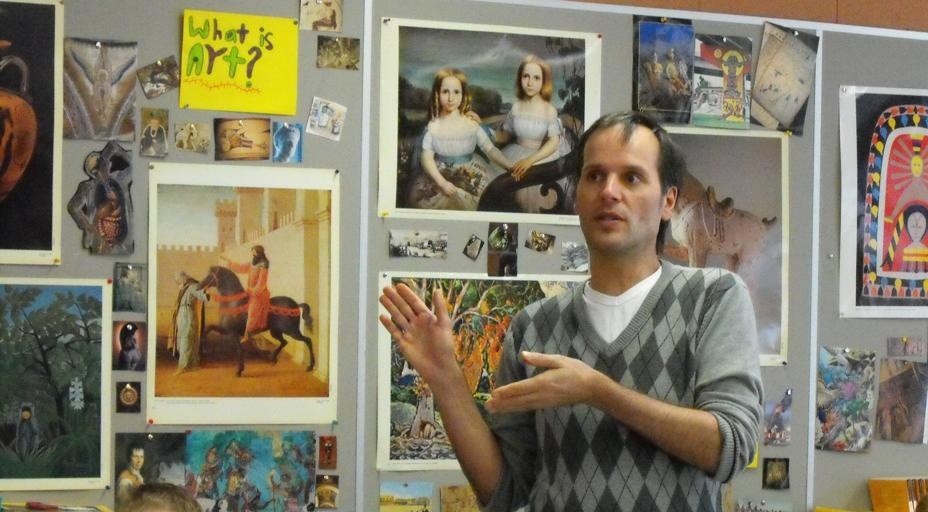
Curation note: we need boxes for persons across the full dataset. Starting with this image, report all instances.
[417,66,513,207]
[166,271,211,377]
[118,479,206,511]
[221,242,274,343]
[372,109,766,510]
[119,439,147,495]
[483,53,565,210]
